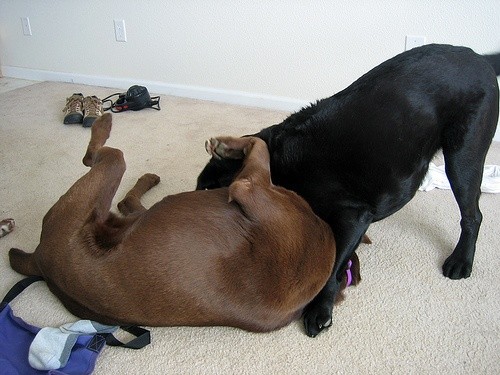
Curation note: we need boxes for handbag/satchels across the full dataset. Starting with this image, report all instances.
[1,271,152,375]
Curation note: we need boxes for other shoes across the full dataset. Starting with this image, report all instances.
[83,94,103,127]
[62,92,85,124]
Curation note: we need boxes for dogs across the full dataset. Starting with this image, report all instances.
[8,42,500,339]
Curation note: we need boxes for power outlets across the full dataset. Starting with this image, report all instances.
[406,36,423,52]
[114,18,126,42]
[21,16,31,35]
